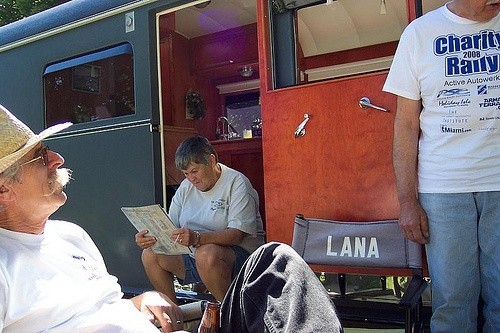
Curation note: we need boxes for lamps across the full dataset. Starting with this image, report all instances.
[379,0,387,15]
[239,66,255,77]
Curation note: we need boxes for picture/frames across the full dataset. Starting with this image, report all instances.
[71,64,101,95]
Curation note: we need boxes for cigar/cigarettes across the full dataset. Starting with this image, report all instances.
[173,234,180,245]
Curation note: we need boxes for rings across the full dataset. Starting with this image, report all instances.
[179,240,182,244]
[176,321,181,324]
[143,243,144,246]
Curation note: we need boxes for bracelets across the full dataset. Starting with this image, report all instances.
[192,231,201,248]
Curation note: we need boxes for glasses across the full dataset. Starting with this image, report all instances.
[11,144,50,170]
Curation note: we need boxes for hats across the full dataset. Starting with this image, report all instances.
[0,104,73,173]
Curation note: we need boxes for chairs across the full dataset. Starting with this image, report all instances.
[288,213,430,333]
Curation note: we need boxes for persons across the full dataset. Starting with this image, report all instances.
[134,137,266,333]
[381,1,500,333]
[0,101,191,333]
